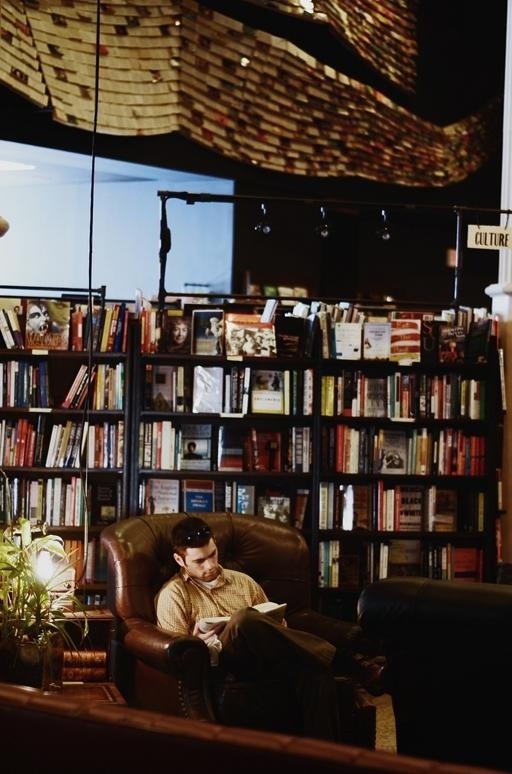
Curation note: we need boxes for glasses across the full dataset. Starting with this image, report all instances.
[183,526,211,547]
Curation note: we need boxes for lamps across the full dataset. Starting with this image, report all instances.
[156,189,512,311]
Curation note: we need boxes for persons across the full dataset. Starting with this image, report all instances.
[156,516,386,743]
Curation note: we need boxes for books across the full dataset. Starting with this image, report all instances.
[250,602,286,623]
[320,480,458,531]
[1,296,129,607]
[387,539,483,581]
[470,492,486,531]
[140,297,320,414]
[139,479,309,528]
[140,421,313,473]
[319,541,387,588]
[322,424,486,475]
[319,303,491,420]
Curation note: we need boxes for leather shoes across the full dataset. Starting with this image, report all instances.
[365,662,390,697]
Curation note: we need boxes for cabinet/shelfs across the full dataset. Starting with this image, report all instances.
[0,293,512,588]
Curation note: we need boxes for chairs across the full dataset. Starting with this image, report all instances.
[357,576,512,772]
[100,511,376,751]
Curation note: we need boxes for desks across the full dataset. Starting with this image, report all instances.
[42,603,116,691]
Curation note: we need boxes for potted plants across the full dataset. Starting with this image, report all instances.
[0,514,89,660]
